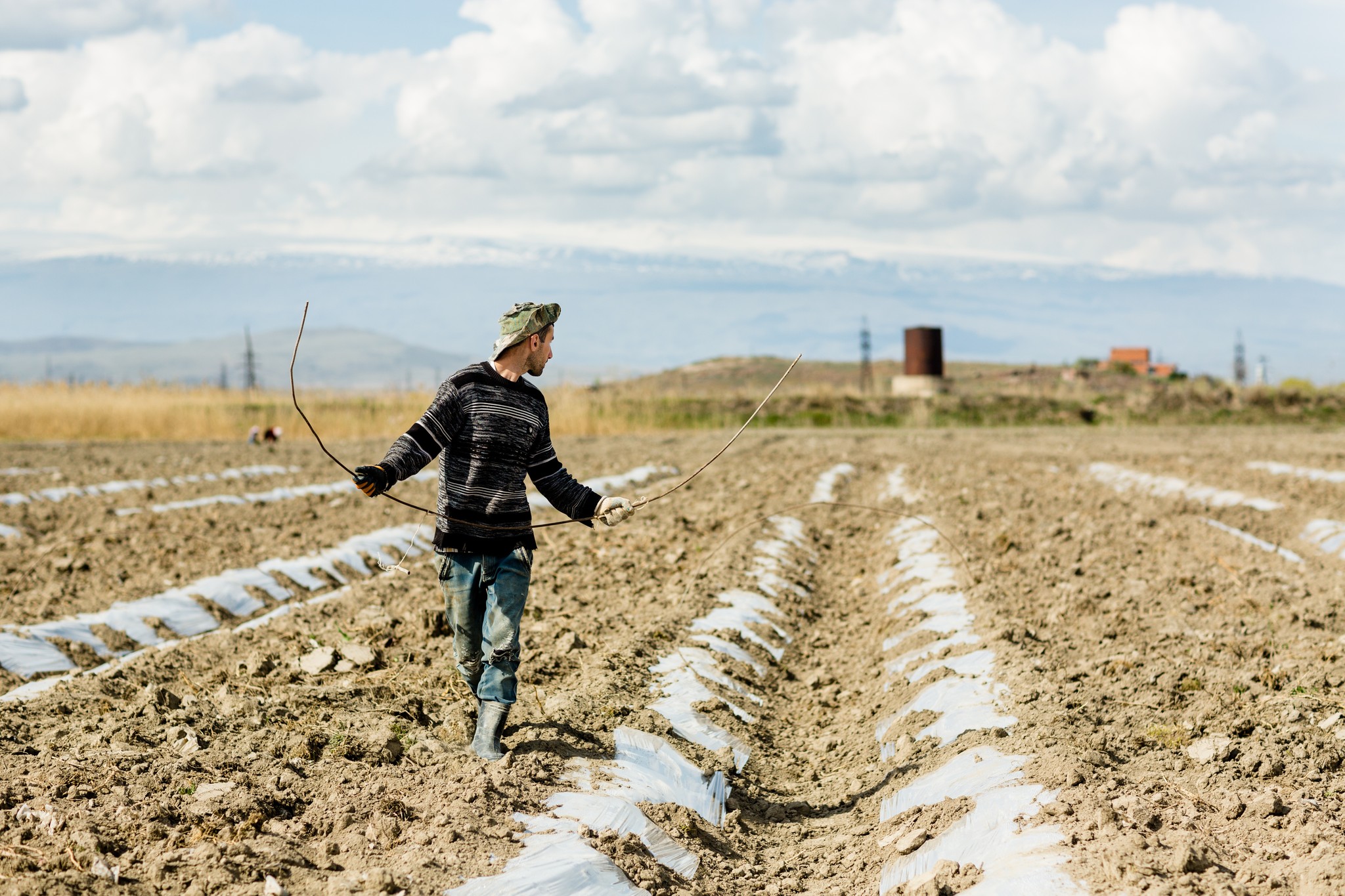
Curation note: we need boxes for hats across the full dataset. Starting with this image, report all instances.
[488,302,561,361]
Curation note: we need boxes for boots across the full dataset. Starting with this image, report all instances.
[468,699,512,761]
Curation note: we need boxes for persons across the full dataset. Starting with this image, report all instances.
[350,303,636,762]
[247,425,282,445]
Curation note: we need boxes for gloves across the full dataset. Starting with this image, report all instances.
[352,465,389,498]
[595,495,635,527]
